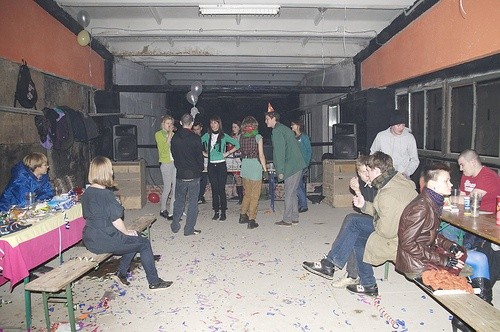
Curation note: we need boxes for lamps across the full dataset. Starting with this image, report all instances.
[199,5,280,14]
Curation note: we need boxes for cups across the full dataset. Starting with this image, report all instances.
[470,193,482,218]
[464,197,471,210]
[451,189,460,207]
[25,192,35,206]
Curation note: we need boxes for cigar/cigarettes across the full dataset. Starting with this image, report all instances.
[364,179,370,187]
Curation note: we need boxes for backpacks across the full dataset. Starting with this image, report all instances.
[13,65,38,110]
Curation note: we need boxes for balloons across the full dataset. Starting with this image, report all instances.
[77,30,90,46]
[78,10,90,28]
[191,81,202,96]
[187,91,198,105]
[149,193,160,203]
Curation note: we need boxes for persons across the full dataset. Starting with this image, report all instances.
[171,113,204,236]
[369,110,420,180]
[395,162,493,332]
[155,115,181,221]
[290,119,312,213]
[229,120,244,205]
[457,150,500,249]
[192,122,207,205]
[0,152,54,282]
[302,151,418,295]
[82,155,173,290]
[264,112,306,227]
[201,115,240,221]
[239,116,269,229]
[331,156,377,288]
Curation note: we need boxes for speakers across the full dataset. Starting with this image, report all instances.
[112,124,138,161]
[332,123,358,159]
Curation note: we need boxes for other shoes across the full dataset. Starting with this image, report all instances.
[198,197,207,203]
[160,209,183,222]
[212,211,226,220]
[29,264,55,282]
[248,219,258,229]
[149,277,172,288]
[184,229,200,236]
[275,220,299,227]
[238,213,249,224]
[115,270,130,285]
[298,207,308,213]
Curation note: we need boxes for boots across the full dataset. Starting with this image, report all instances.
[452,277,494,332]
[235,185,243,204]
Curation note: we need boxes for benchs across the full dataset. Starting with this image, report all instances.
[25,216,157,332]
[384,258,500,332]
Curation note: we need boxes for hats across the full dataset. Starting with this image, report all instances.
[389,109,405,125]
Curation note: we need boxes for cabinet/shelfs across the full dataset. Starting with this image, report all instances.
[322,159,358,207]
[110,159,146,209]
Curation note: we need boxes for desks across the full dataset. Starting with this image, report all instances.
[0,195,85,332]
[440,204,500,246]
[202,170,278,210]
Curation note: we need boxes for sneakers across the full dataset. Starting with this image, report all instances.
[302,254,333,280]
[346,281,378,297]
[331,271,360,288]
